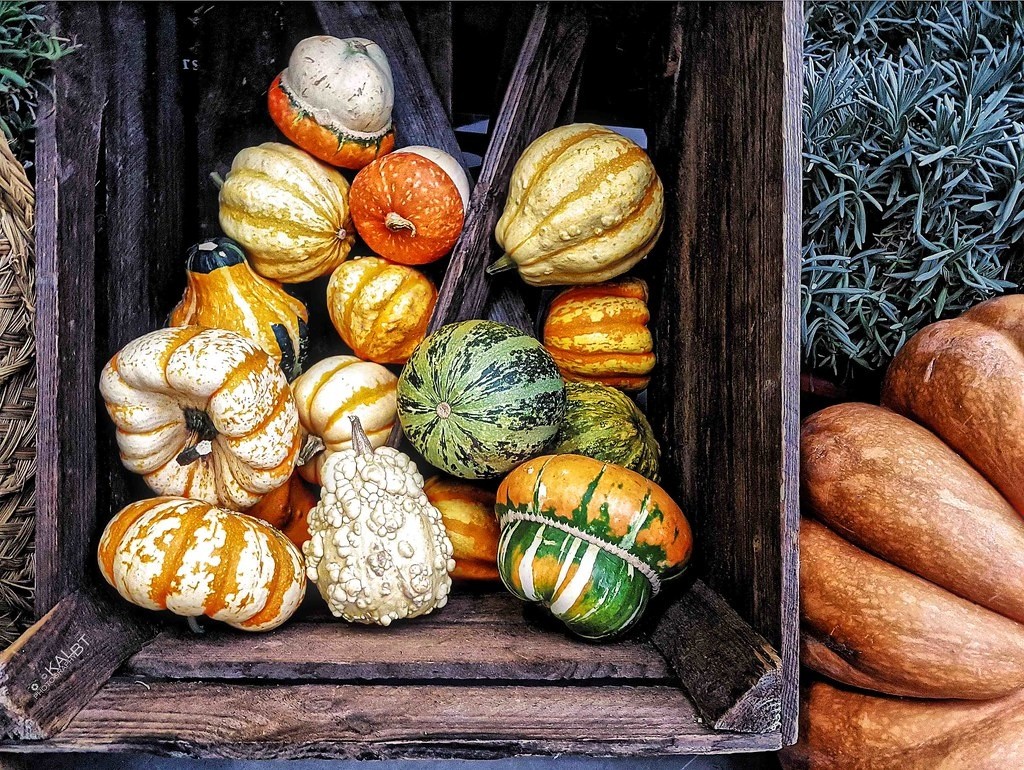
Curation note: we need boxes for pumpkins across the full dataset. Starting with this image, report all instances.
[96,35,692,642]
[798,294,1024,770]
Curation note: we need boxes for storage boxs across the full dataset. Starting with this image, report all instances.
[0,0,809,761]
